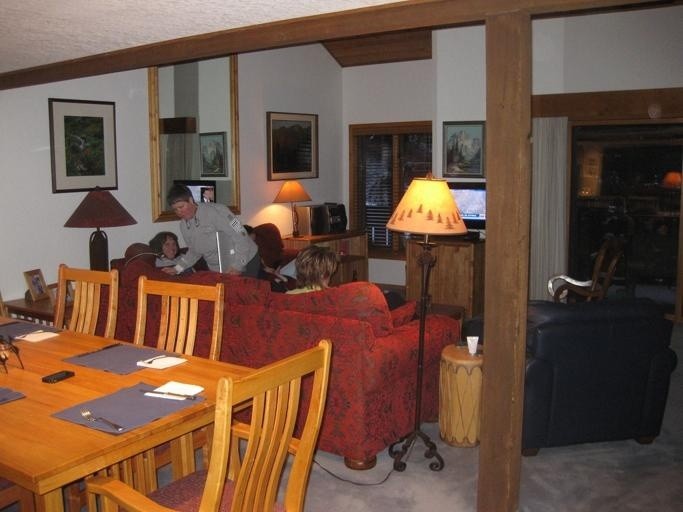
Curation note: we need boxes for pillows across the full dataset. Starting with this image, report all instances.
[391,301,416,325]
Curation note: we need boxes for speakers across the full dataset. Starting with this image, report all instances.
[324,202,348,233]
[294,204,331,236]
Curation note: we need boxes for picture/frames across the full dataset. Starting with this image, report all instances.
[266,110,319,182]
[199,132,228,177]
[47,280,75,311]
[48,97,118,193]
[443,120,485,178]
[23,268,51,302]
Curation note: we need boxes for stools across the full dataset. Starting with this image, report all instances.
[441,341,485,448]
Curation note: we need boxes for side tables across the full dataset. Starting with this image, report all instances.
[4,288,77,330]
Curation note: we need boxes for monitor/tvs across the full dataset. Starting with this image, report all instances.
[447,181,486,232]
[173,179,217,209]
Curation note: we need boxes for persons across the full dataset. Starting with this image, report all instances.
[202,189,213,203]
[162,185,261,279]
[243,224,288,282]
[285,246,338,295]
[151,231,197,276]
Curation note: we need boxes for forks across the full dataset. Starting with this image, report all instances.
[142,356,167,365]
[78,407,123,432]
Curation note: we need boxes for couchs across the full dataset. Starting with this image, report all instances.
[520,298,677,458]
[95,242,460,471]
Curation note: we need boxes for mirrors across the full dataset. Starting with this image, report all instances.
[148,54,241,223]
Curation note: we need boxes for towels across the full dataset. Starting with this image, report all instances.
[143,380,205,401]
[137,355,187,370]
[14,329,60,343]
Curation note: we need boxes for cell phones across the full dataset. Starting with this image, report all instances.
[41,369,75,384]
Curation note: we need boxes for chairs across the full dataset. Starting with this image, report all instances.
[133,275,224,495]
[547,236,624,304]
[87,340,331,512]
[55,263,118,339]
[0,485,35,512]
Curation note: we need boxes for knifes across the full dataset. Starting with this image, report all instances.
[139,388,196,401]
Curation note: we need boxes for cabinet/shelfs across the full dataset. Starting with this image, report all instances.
[405,238,485,318]
[278,230,368,287]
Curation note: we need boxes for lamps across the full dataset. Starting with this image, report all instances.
[63,186,137,272]
[272,180,311,238]
[387,170,466,473]
